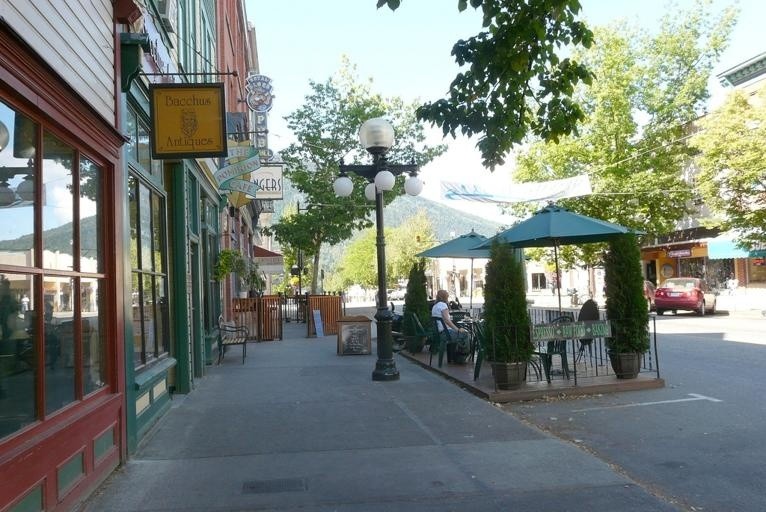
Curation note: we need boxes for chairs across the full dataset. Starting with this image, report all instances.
[533,315,572,384]
[411,311,487,381]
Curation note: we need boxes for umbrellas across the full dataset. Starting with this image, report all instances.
[467,200,648,316]
[413,228,532,309]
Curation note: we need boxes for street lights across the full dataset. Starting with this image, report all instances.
[332,117,423,381]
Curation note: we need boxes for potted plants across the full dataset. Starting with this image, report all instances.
[603,232,650,378]
[482,236,534,391]
[403,258,431,353]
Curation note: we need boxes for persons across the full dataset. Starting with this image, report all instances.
[432,289,468,363]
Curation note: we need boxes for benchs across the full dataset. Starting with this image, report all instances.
[217,314,250,365]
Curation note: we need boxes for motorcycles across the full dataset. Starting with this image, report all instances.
[389,296,462,353]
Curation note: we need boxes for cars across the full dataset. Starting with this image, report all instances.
[643,280,657,313]
[386,288,404,301]
[654,277,717,316]
